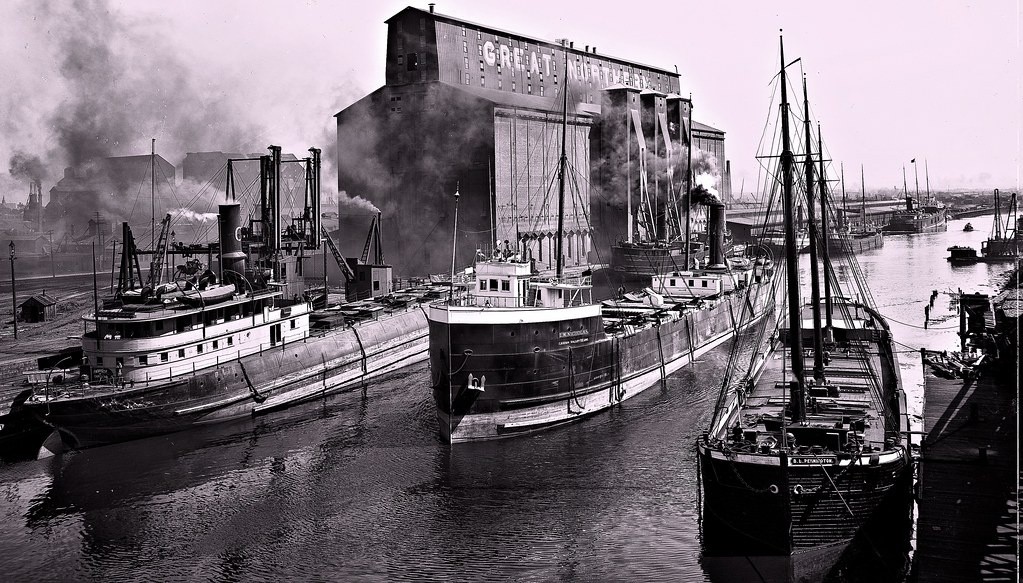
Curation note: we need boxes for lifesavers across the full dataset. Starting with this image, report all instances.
[551,277,559,287]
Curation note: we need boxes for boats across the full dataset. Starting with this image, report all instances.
[889,158,948,231]
[124,281,177,297]
[23,143,455,444]
[688,30,911,583]
[428,47,776,443]
[947,245,978,263]
[963,222,974,231]
[177,284,236,303]
[820,161,885,254]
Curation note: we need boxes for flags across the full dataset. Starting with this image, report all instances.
[911,159,915,163]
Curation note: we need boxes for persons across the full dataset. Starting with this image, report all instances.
[115,361,123,386]
[732,420,744,444]
[618,285,627,301]
[293,290,316,312]
[103,330,122,340]
[140,281,155,305]
